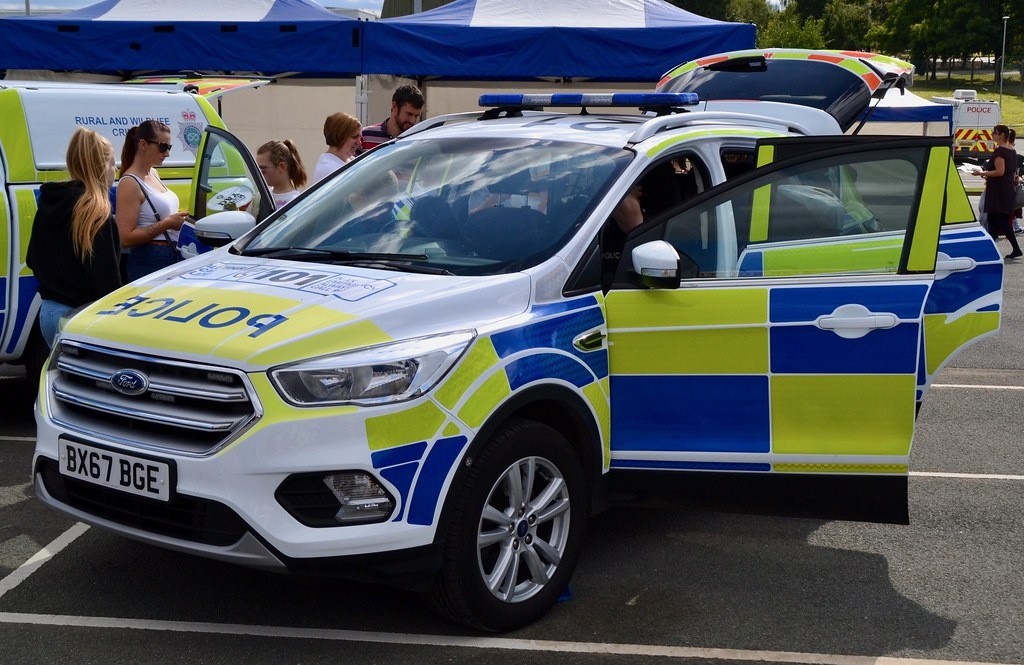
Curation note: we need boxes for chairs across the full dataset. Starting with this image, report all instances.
[465,156,550,246]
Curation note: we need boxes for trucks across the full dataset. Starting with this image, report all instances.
[932,89,1003,163]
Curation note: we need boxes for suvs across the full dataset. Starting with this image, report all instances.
[31,48,1003,633]
[0,80,257,392]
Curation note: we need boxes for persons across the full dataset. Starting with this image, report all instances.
[238,139,309,234]
[591,155,699,290]
[312,169,400,245]
[115,120,190,282]
[972,123,1024,258]
[354,83,428,187]
[25,129,123,352]
[307,113,363,184]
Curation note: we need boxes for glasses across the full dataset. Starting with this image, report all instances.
[137,139,172,152]
[992,131,1001,135]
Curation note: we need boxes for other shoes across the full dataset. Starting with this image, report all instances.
[1005,251,1022,258]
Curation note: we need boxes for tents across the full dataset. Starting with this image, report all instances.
[0,0,758,214]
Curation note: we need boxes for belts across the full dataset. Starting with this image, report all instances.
[150,242,171,246]
